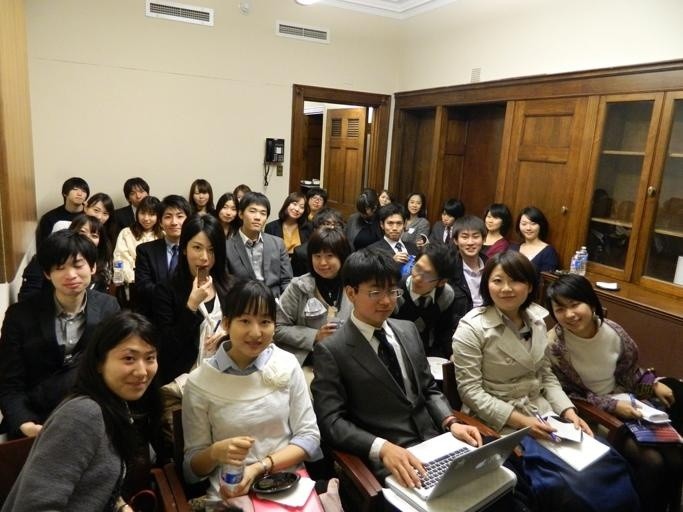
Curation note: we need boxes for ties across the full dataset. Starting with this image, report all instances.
[168,244,178,278]
[395,243,402,252]
[443,226,450,245]
[372,327,406,397]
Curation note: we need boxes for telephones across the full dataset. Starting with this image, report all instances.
[264,137,284,185]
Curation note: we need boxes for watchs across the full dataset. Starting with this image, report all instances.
[444,418,458,430]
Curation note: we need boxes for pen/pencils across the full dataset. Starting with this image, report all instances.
[211,320,221,335]
[630,393,641,427]
[537,412,556,439]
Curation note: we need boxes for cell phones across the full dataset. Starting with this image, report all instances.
[197,267,207,287]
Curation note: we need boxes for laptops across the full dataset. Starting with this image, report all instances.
[406,427,532,500]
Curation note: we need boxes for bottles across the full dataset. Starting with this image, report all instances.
[567,251,580,274]
[578,246,588,277]
[112,252,124,284]
[219,463,244,490]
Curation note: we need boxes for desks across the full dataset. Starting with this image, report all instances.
[536,267,683,382]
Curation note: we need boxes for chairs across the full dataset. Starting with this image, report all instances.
[345,410,501,494]
[165,408,379,512]
[1,437,177,511]
[441,362,621,455]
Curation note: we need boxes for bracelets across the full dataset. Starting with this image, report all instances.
[259,460,268,477]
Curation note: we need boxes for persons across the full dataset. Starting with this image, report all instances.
[291,207,351,277]
[182,277,324,511]
[214,192,239,238]
[82,193,114,227]
[348,189,383,250]
[133,194,192,318]
[264,191,312,255]
[365,203,419,281]
[153,214,240,370]
[113,177,150,226]
[544,273,682,512]
[35,177,89,253]
[113,196,166,304]
[273,226,351,371]
[233,184,251,203]
[223,192,294,298]
[17,214,106,302]
[0,229,121,438]
[451,248,636,512]
[309,246,484,512]
[189,179,215,215]
[506,206,558,299]
[428,198,465,243]
[354,205,406,248]
[378,189,394,206]
[478,203,510,260]
[444,213,491,318]
[0,309,159,512]
[390,244,468,390]
[403,193,431,243]
[305,187,327,220]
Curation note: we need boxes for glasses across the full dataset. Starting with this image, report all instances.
[414,264,440,282]
[312,195,324,202]
[357,289,401,301]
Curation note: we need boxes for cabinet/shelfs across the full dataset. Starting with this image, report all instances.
[388,59,683,300]
